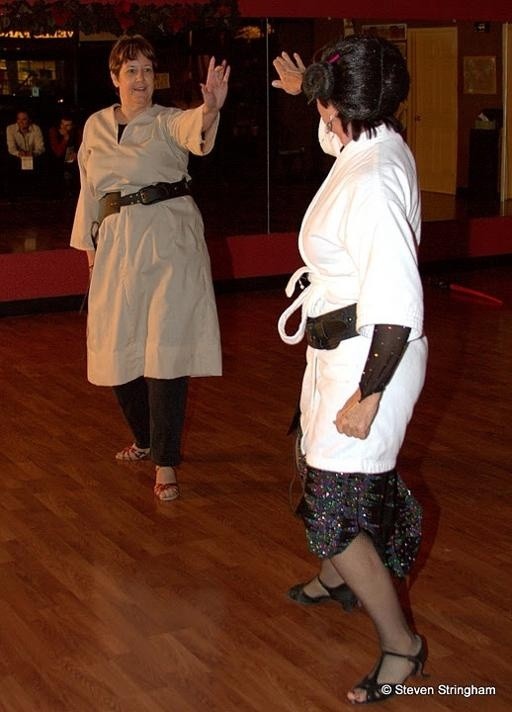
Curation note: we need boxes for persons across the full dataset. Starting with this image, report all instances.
[49,113,83,201]
[74,32,232,500]
[267,28,433,702]
[6,108,47,200]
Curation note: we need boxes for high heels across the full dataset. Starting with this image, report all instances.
[287,572,359,611]
[348,633,428,704]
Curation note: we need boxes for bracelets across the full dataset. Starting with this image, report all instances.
[89,265,94,269]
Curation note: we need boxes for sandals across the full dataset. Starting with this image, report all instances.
[115,442,152,461]
[154,465,180,501]
